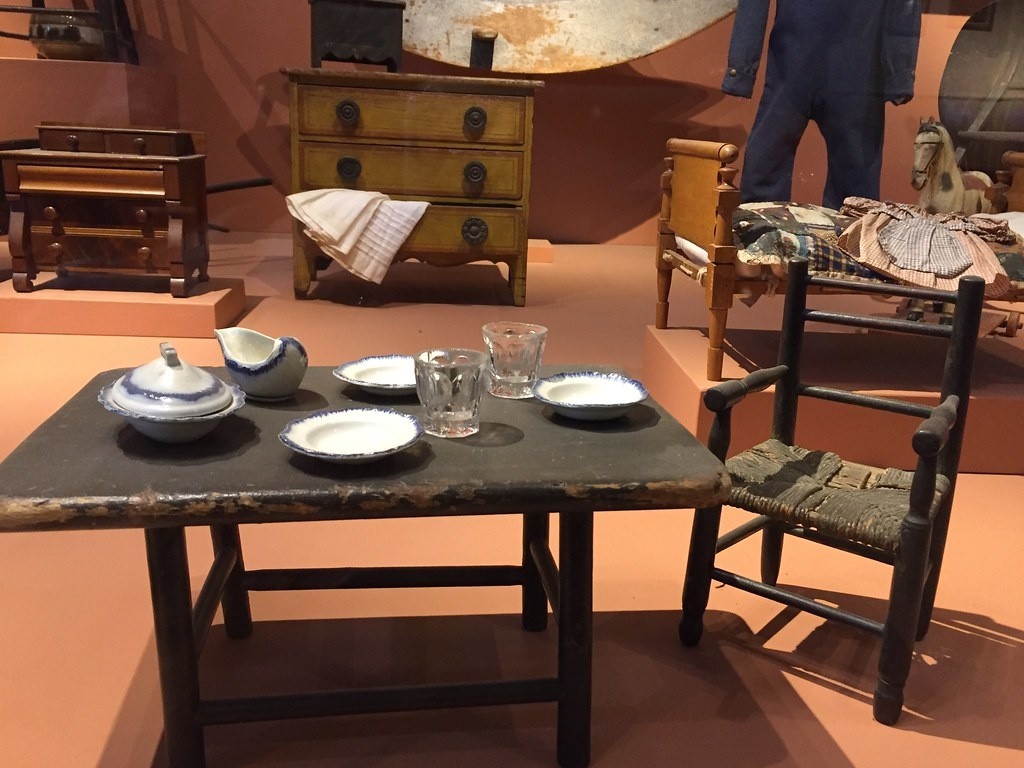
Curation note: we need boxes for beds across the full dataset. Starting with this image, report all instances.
[657,137,1024,379]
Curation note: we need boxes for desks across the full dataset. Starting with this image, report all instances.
[0,360,734,767]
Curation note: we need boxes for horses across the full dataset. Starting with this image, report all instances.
[909,115,993,216]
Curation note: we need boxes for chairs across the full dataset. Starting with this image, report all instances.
[677,259,985,723]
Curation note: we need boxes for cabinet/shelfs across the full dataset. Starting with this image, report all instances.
[1,125,210,299]
[278,64,545,304]
